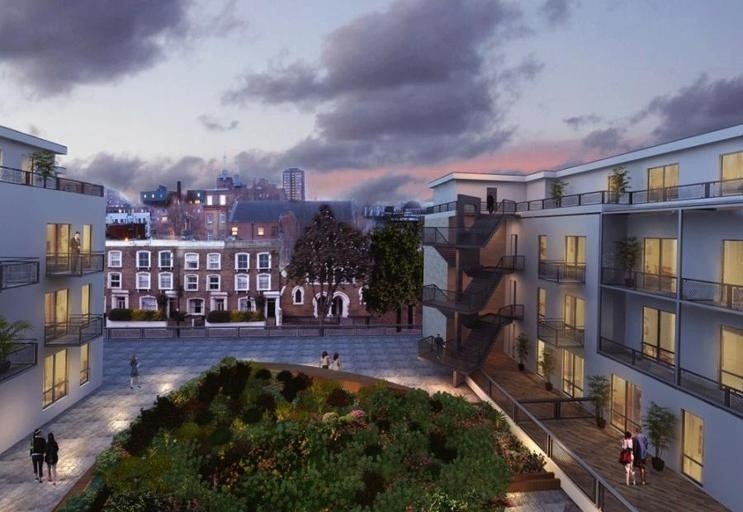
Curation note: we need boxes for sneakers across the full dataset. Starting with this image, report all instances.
[34,478,56,486]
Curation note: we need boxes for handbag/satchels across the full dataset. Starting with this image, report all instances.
[620,450,631,464]
[30,448,34,456]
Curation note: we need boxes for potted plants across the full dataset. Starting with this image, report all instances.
[512,332,530,372]
[1,316,33,375]
[614,235,642,287]
[641,400,680,473]
[536,350,557,393]
[585,372,614,429]
